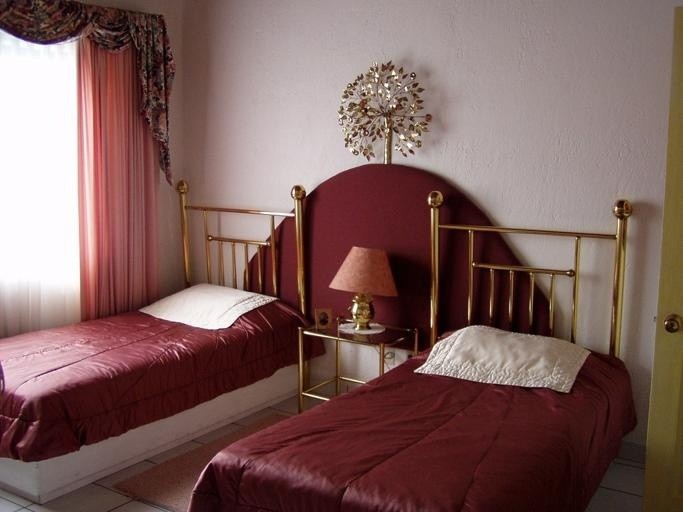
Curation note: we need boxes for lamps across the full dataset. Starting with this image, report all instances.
[328,246,398,334]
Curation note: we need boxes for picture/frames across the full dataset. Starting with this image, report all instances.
[315,308,332,330]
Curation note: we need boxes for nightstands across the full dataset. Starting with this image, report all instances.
[298,317,418,414]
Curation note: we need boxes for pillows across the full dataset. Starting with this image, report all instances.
[141,283,278,330]
[413,324,591,393]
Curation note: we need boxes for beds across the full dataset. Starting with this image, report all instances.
[188,191,632,510]
[1,181,310,505]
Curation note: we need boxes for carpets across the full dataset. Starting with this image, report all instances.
[114,415,292,512]
